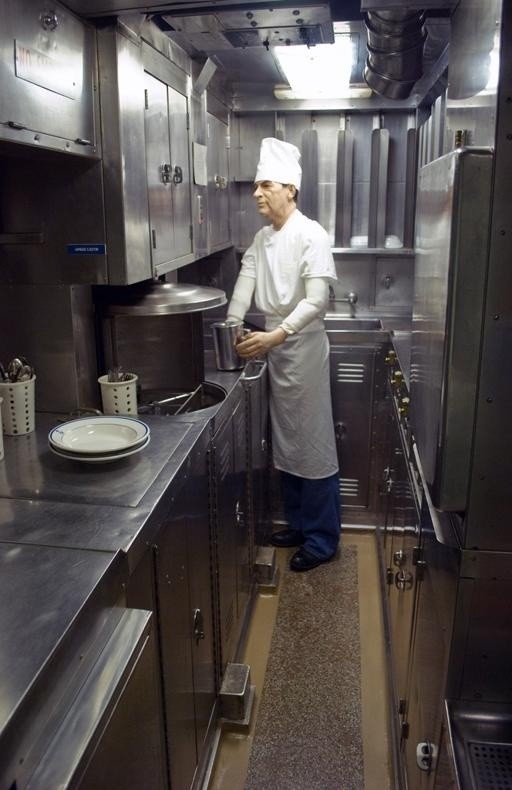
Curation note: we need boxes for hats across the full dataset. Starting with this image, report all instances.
[254,136,303,186]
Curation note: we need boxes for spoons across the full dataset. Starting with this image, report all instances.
[0,355,34,383]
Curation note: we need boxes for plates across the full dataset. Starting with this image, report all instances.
[46,415,151,467]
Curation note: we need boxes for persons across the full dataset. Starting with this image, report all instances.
[224,133,341,574]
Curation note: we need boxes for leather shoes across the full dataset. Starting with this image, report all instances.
[269,530,302,548]
[290,550,321,572]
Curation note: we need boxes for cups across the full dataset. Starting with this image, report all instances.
[209,319,252,371]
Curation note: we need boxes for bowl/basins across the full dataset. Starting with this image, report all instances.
[363,5,431,102]
